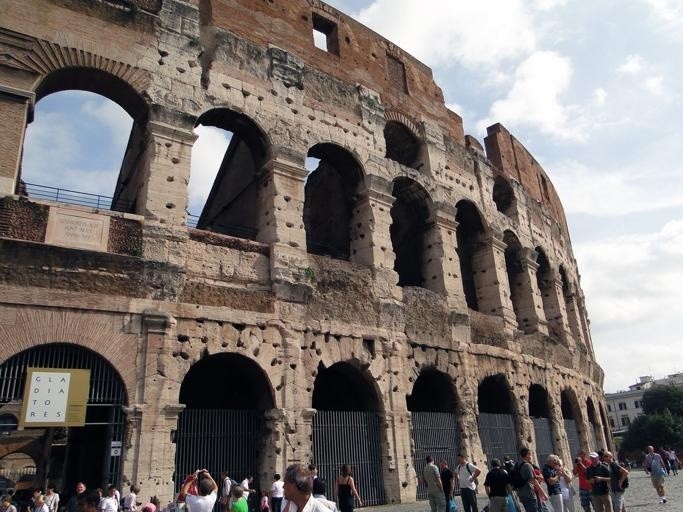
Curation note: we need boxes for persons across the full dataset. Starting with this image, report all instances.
[0,462,364,512]
[422,446,683,511]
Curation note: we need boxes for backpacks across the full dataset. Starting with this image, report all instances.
[511,462,529,488]
[459,463,479,486]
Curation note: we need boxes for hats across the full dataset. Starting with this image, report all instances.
[589,452,598,458]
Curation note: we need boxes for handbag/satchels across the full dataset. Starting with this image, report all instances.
[178,475,197,500]
[646,463,651,472]
[617,475,629,488]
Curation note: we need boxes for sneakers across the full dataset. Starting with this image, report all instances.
[659,497,667,504]
